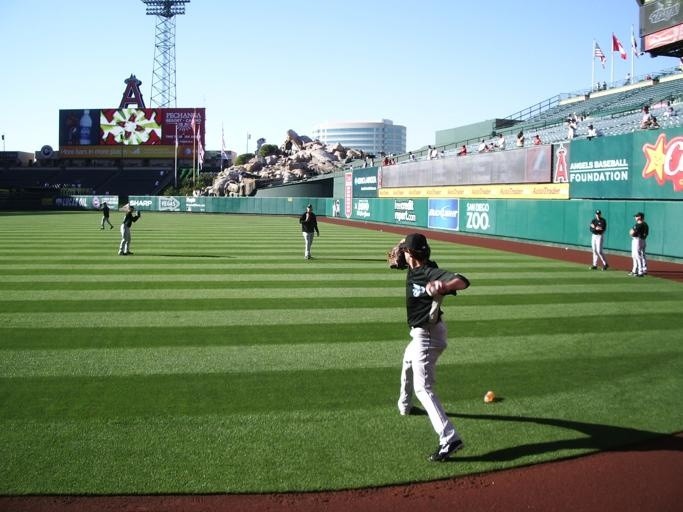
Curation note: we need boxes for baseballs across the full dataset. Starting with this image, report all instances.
[430,287,437,295]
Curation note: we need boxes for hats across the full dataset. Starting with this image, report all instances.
[634,212,644,217]
[596,210,601,215]
[400,233,427,251]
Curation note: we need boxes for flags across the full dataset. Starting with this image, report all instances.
[631,32,638,56]
[595,42,606,68]
[613,34,627,60]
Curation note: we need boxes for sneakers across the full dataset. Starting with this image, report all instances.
[590,265,608,271]
[427,440,464,462]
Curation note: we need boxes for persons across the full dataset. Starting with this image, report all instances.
[663,101,675,117]
[457,145,466,156]
[384,157,395,165]
[299,204,319,259]
[428,145,445,160]
[363,157,374,167]
[589,209,608,271]
[118,206,140,255]
[478,134,505,153]
[534,134,541,145]
[100,202,113,229]
[409,152,416,160]
[587,124,602,140]
[387,233,470,461]
[517,131,524,147]
[567,113,590,139]
[627,213,649,277]
[640,105,660,130]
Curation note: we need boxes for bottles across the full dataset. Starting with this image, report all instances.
[79,109,93,145]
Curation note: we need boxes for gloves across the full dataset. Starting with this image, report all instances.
[425,280,447,297]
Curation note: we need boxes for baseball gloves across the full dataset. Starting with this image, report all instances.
[386,243,408,270]
